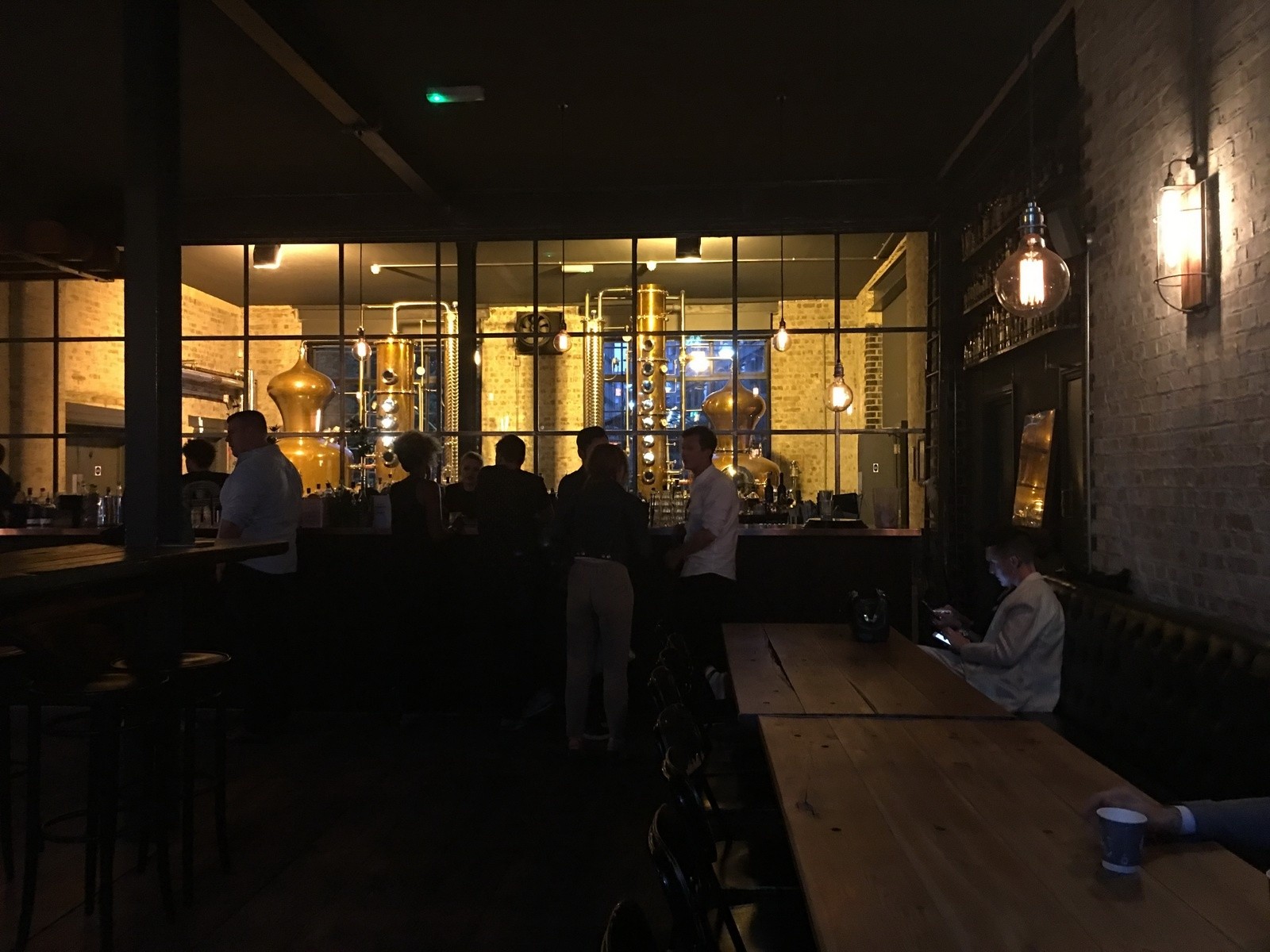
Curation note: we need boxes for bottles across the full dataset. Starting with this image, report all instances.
[300,474,395,497]
[539,473,557,497]
[734,471,800,516]
[11,480,124,525]
[430,472,450,487]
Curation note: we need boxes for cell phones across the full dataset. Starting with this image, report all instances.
[932,609,953,615]
[932,631,951,646]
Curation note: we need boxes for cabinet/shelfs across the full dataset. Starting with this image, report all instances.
[961,178,1084,368]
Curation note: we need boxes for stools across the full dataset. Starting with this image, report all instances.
[0,647,231,952]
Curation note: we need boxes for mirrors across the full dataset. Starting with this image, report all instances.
[1011,406,1059,534]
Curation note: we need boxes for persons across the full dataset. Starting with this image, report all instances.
[473,434,558,732]
[211,411,303,743]
[387,433,467,733]
[0,444,16,528]
[446,451,483,522]
[558,427,608,502]
[554,444,651,765]
[1089,789,1270,849]
[933,533,1066,714]
[176,439,232,539]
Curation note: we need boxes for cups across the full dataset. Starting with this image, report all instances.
[1095,806,1148,874]
[753,490,836,523]
[650,490,690,528]
[190,505,223,529]
[856,598,889,642]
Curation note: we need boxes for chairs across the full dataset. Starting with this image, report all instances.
[602,621,820,952]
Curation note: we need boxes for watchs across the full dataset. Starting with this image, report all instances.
[663,425,739,705]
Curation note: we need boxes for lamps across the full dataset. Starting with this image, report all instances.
[676,237,702,259]
[253,244,281,267]
[1154,151,1219,315]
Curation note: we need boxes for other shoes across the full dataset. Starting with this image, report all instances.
[605,749,628,766]
[559,747,581,757]
[224,726,270,744]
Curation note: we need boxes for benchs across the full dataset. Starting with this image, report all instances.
[997,576,1270,874]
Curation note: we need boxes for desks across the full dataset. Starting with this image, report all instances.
[756,714,1270,952]
[0,537,287,599]
[723,623,1015,720]
